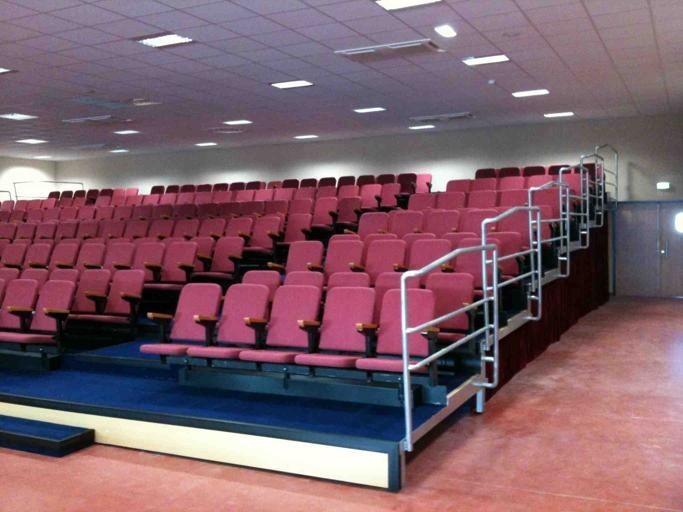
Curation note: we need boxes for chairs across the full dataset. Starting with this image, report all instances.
[0,163,633,388]
